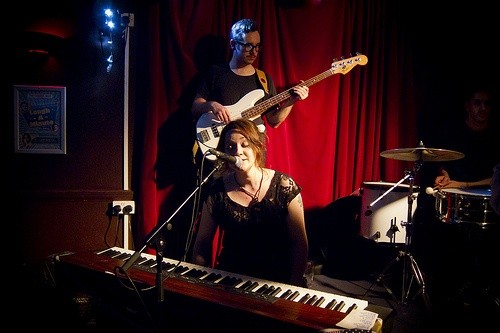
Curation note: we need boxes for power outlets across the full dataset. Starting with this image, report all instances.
[112,201,135,215]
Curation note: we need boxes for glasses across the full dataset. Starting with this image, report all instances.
[235,40,262,52]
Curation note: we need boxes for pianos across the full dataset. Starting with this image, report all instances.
[53,246,383,333]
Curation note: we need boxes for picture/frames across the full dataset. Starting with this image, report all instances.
[14,85,67,155]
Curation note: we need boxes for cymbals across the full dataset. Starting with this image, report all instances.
[380,147,464,163]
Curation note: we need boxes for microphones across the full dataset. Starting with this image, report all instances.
[209,150,244,170]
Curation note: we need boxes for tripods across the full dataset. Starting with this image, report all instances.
[359,169,432,316]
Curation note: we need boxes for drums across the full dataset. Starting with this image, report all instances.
[363,181,420,244]
[436,185,495,229]
[321,190,371,276]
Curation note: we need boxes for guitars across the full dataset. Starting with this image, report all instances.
[196,51,368,162]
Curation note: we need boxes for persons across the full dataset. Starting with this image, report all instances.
[186,19,309,266]
[194,120,307,333]
[412,85,500,252]
